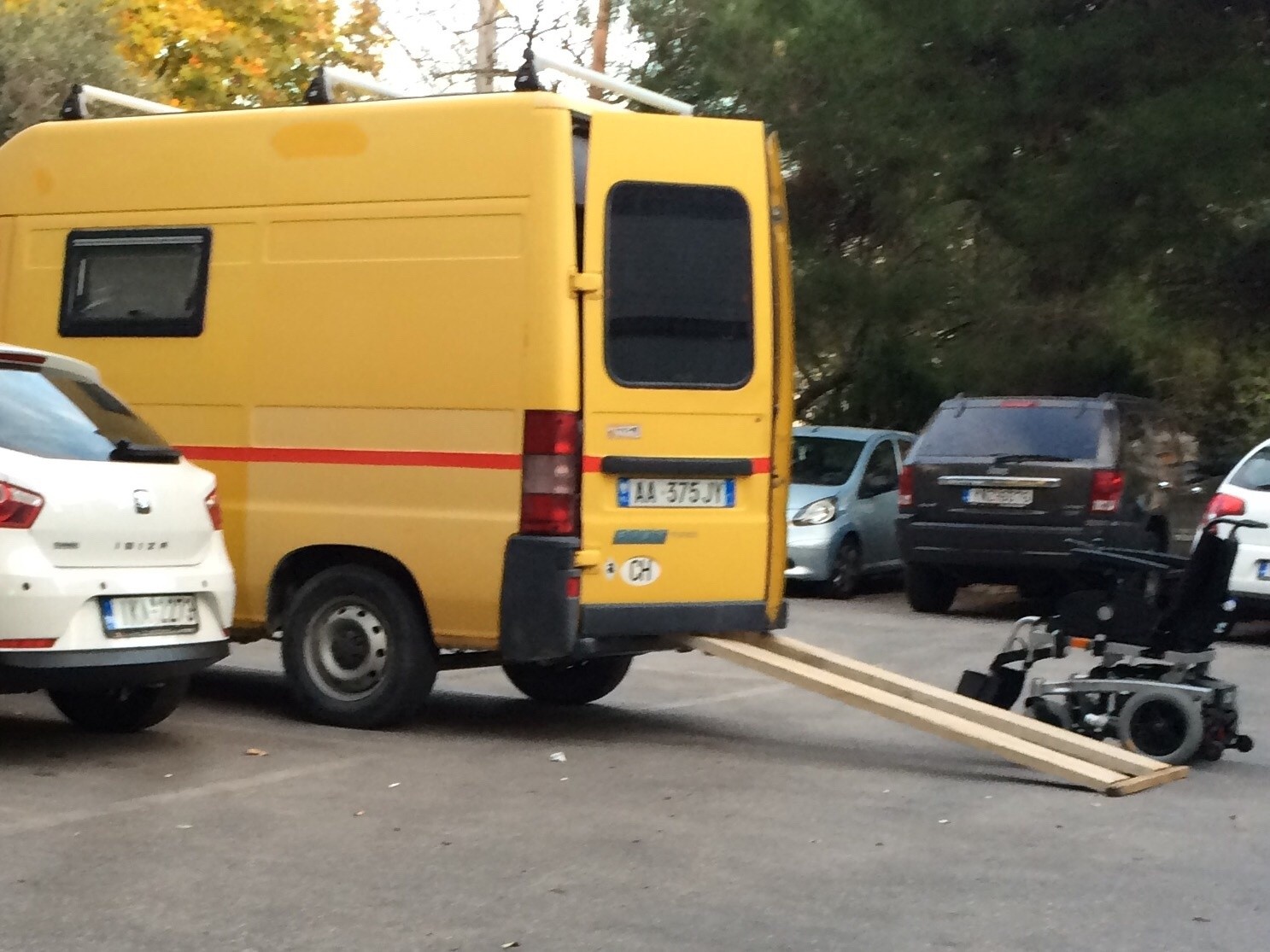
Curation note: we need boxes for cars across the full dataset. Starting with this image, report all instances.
[1187,439,1270,643]
[782,426,920,601]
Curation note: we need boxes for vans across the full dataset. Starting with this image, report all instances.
[1,49,797,729]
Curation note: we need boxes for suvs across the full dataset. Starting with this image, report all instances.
[0,340,238,737]
[893,390,1219,627]
